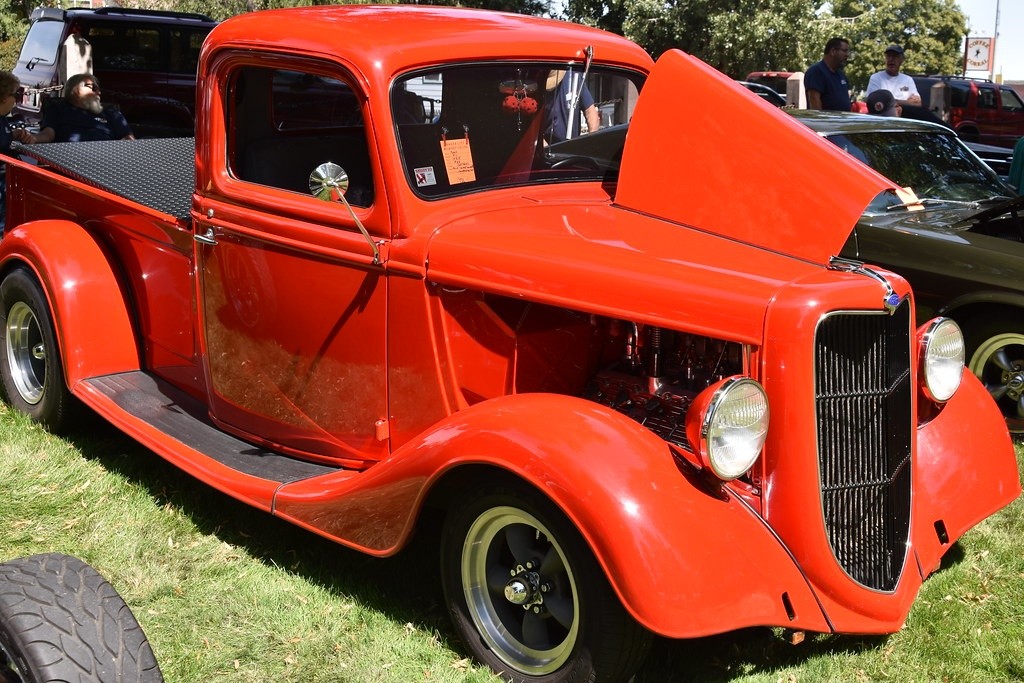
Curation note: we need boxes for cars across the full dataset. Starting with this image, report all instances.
[532,105,1024,447]
[0,0,1022,683]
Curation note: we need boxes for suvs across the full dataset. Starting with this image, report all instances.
[12,3,431,167]
[740,72,798,108]
[914,70,1024,155]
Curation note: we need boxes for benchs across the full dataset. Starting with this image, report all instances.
[243,124,440,189]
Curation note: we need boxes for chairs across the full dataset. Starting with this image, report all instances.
[38,97,120,132]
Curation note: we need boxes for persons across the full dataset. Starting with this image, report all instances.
[0,70,27,235]
[866,89,954,131]
[544,71,600,144]
[866,45,922,106]
[20,74,136,145]
[804,38,851,112]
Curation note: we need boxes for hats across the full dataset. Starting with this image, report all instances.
[866,89,896,115]
[884,45,903,55]
[546,69,567,90]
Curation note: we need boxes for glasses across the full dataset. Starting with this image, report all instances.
[835,47,850,55]
[85,82,101,92]
[8,91,21,101]
